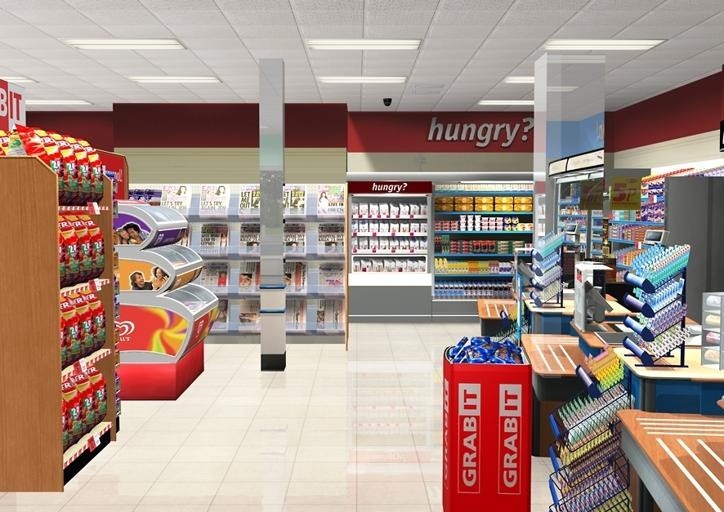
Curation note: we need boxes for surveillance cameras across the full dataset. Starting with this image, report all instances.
[383,98,392,106]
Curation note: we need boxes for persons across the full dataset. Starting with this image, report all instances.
[117,222,169,290]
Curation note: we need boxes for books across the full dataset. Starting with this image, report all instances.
[160,184,345,330]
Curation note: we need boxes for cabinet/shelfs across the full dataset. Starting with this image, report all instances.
[188,212,346,343]
[701,292,724,370]
[117,204,219,400]
[351,193,428,272]
[432,190,533,302]
[560,213,602,255]
[624,269,688,367]
[1,155,116,492]
[608,219,665,270]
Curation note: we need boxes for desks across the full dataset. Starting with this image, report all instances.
[613,348,724,414]
[616,408,724,512]
[522,290,618,318]
[477,298,518,337]
[524,299,630,334]
[522,334,598,457]
